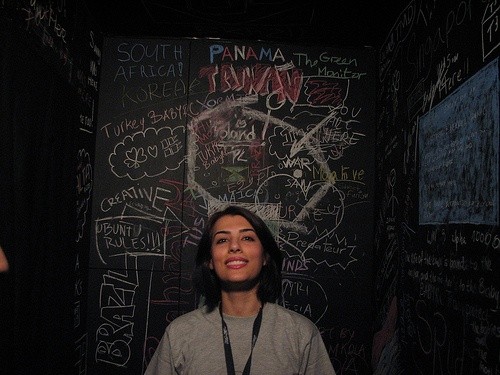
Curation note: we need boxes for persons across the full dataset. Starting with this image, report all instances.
[144,206,336,375]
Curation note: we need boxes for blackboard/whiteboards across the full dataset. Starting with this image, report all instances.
[0,0,101,374]
[376,1,500,375]
[87,34,377,375]
